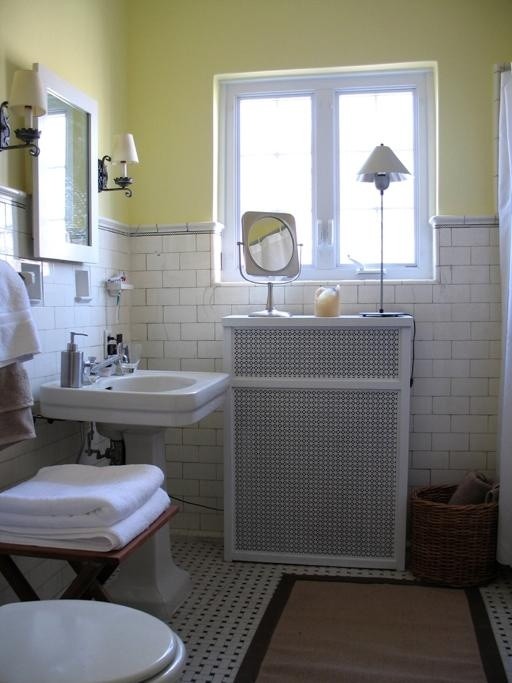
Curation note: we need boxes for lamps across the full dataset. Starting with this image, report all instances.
[355,144,412,317]
[0,70,48,158]
[98,133,141,198]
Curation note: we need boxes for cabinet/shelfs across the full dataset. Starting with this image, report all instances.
[219,313,415,571]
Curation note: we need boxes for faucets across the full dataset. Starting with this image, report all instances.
[90,353,128,374]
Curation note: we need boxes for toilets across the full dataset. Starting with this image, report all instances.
[2,600,187,683]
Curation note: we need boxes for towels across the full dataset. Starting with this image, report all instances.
[0,463,172,554]
[0,258,42,452]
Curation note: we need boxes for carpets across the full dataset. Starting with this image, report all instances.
[233,572,510,683]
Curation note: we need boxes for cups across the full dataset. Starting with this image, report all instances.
[116,342,143,374]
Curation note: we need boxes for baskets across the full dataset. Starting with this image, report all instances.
[409,483,499,588]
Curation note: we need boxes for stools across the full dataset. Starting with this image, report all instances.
[0,504,180,603]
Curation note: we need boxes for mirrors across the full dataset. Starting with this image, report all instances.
[31,61,100,265]
[237,210,303,317]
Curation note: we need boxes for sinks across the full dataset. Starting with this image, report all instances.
[41,367,230,427]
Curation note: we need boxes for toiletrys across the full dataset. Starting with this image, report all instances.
[97,361,113,377]
